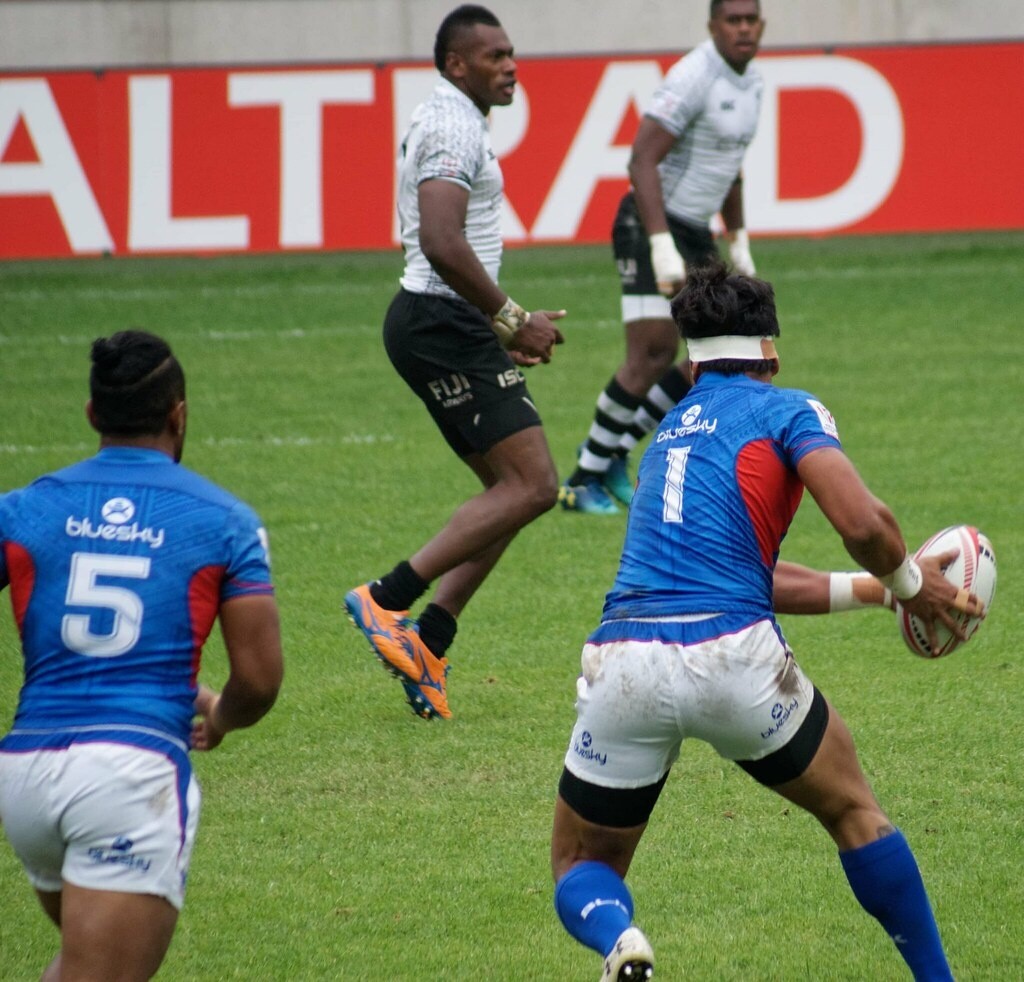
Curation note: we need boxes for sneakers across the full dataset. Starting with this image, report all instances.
[576,439,636,508]
[340,579,422,684]
[599,926,656,982]
[557,478,619,514]
[399,627,453,721]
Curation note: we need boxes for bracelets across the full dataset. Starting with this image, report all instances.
[490,295,529,345]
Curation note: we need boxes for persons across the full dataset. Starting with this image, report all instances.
[345,5,567,716]
[552,270,986,982]
[0,332,282,981]
[557,0,765,514]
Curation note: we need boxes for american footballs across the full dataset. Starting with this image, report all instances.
[901,519,999,658]
